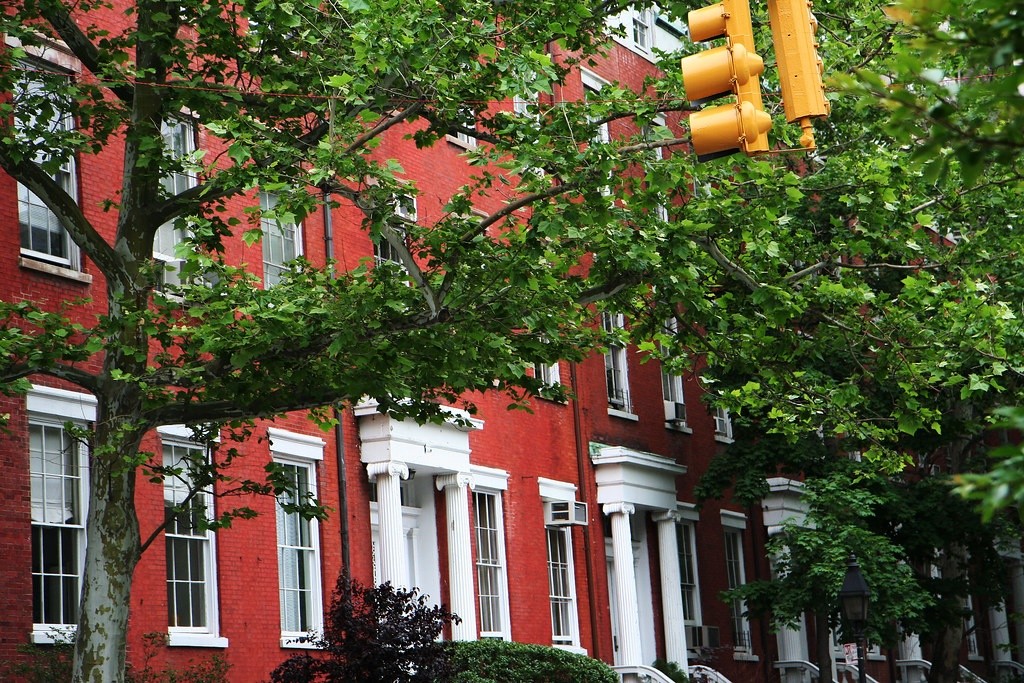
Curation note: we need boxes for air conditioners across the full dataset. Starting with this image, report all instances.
[713,417,725,434]
[664,401,686,422]
[385,192,418,224]
[684,625,718,650]
[162,260,204,291]
[543,500,588,526]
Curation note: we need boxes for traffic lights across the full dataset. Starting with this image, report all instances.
[681,0,771,160]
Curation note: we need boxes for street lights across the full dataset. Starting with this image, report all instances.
[842,552,871,683]
